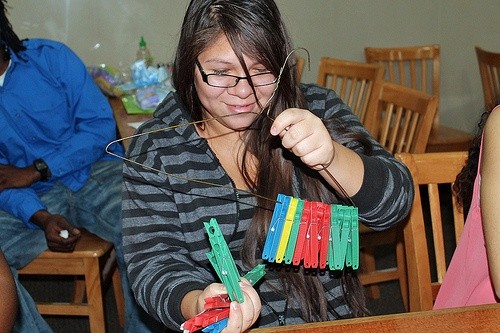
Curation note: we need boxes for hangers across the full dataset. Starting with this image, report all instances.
[104,48,359,218]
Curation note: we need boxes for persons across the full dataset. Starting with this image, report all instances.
[431,104,499,310]
[0,0,171,332]
[121,0,416,333]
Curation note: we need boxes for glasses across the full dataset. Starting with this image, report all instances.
[195,57,279,88]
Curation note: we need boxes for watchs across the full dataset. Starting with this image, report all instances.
[34,158,49,181]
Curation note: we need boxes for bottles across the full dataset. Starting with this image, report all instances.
[136,35,153,67]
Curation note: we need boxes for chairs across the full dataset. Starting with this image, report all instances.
[350,79,439,312]
[13,94,126,333]
[316,55,385,135]
[474,46,500,112]
[362,44,478,229]
[393,151,473,312]
